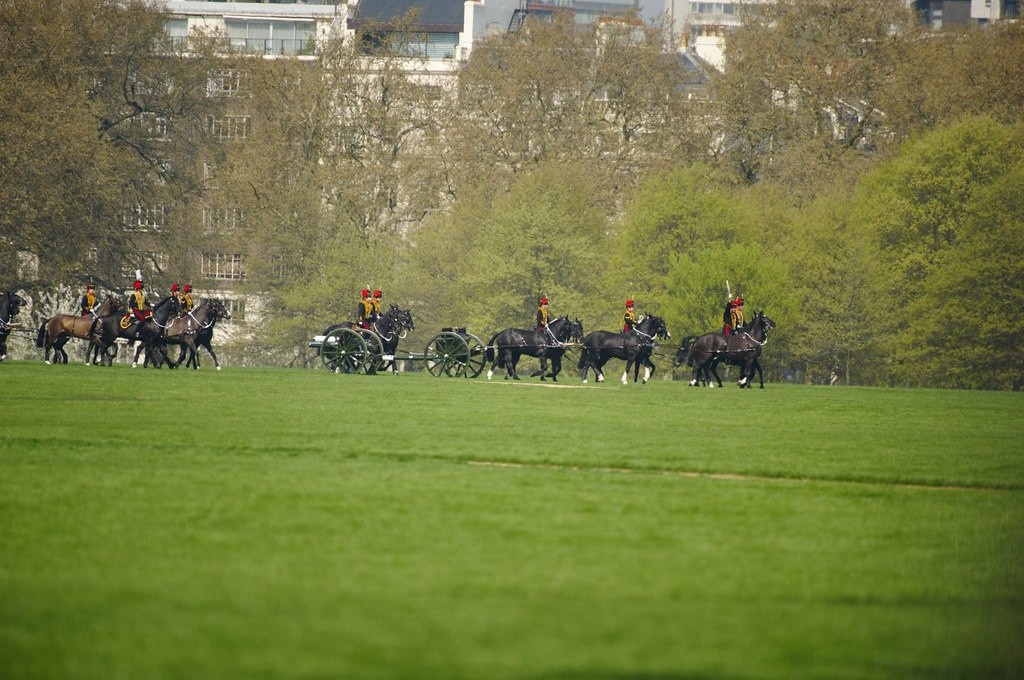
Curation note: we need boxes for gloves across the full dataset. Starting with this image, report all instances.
[544,324,548,327]
[729,293,733,303]
[638,321,641,324]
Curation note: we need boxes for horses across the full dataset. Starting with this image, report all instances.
[0,285,232,370]
[669,310,777,389]
[483,310,671,385]
[316,303,415,375]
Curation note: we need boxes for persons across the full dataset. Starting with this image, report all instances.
[357,283,384,351]
[721,296,746,336]
[180,285,194,318]
[170,283,181,298]
[80,285,99,316]
[126,280,155,347]
[533,297,552,330]
[623,300,642,334]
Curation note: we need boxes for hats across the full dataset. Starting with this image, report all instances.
[626,294,635,307]
[727,300,734,308]
[86,277,95,289]
[541,294,550,304]
[735,293,744,306]
[184,280,192,291]
[134,269,144,288]
[373,286,382,297]
[171,279,180,291]
[361,284,371,296]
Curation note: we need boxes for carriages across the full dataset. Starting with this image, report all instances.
[308,305,777,390]
